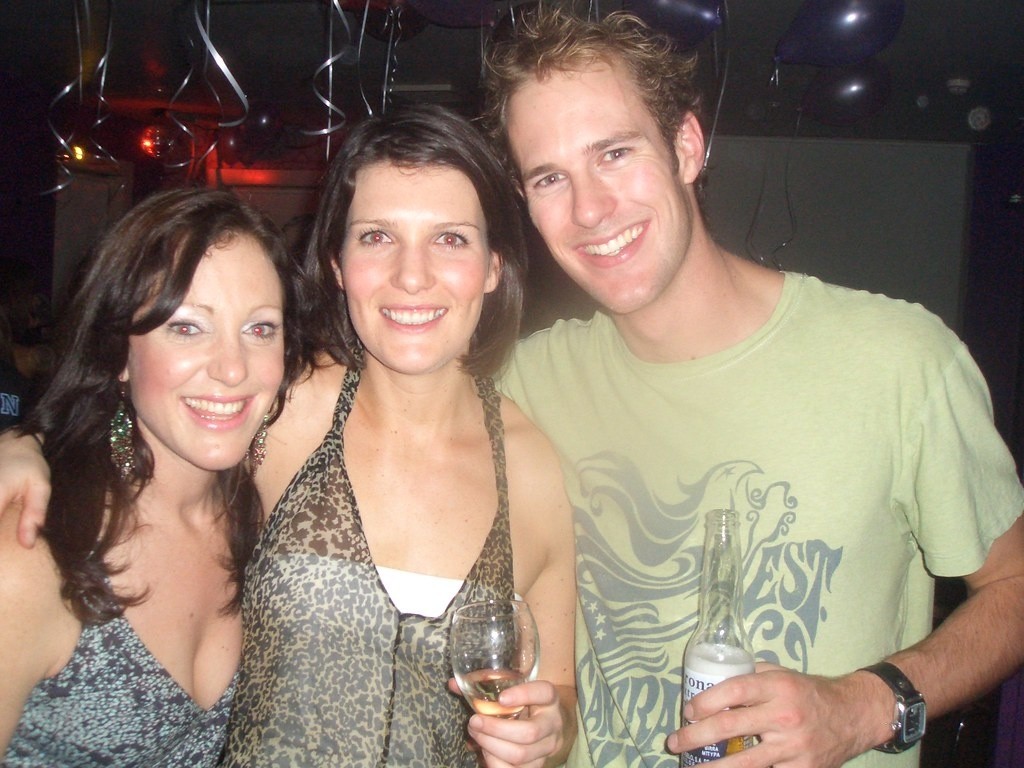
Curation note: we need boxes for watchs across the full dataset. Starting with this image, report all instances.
[857,662,927,754]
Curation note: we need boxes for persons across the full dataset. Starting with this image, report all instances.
[0,108,577,768]
[492,0,1024,768]
[0,188,307,768]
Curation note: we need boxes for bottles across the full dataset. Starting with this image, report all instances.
[678,511,756,768]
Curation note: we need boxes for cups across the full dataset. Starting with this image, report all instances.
[451,598,541,723]
